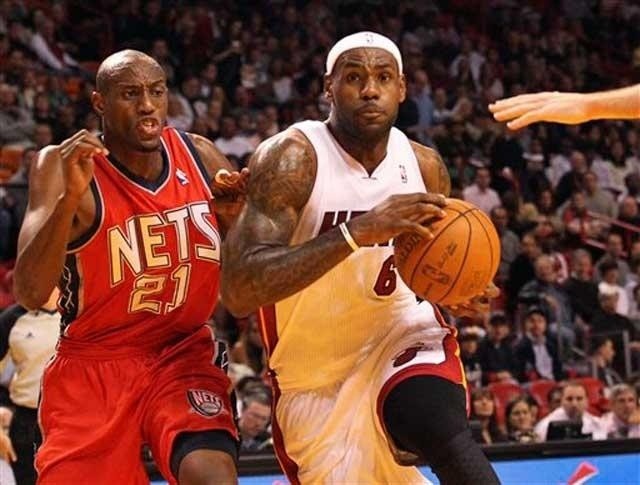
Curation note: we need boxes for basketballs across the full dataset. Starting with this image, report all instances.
[393,198,501,306]
